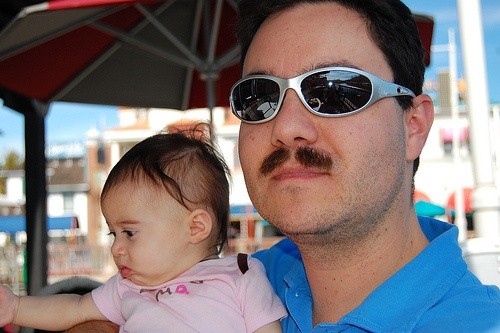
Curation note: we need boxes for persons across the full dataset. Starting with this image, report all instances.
[65,0,500,333]
[0,131,288,333]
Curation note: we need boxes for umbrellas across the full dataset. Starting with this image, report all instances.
[0,0,433,151]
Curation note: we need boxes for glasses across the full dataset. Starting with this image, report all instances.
[229,66,416,123]
